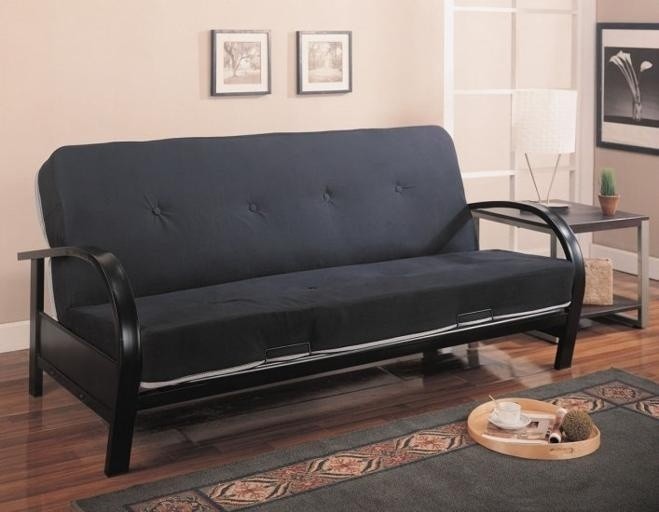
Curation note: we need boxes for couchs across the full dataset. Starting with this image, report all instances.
[17,124,586,481]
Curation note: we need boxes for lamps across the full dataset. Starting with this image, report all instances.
[512,90,578,212]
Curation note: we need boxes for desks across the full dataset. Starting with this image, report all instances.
[471,197,650,341]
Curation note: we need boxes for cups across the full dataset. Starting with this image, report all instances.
[496,401,522,426]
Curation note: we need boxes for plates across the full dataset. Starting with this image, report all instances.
[487,413,531,431]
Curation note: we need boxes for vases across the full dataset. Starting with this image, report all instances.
[631,102,643,124]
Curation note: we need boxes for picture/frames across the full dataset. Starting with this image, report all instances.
[210,29,272,97]
[596,23,659,154]
[297,30,354,94]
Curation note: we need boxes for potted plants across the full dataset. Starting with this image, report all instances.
[597,166,621,215]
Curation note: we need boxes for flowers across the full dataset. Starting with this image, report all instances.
[606,50,654,119]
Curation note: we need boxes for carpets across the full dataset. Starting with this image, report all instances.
[71,364,658,512]
